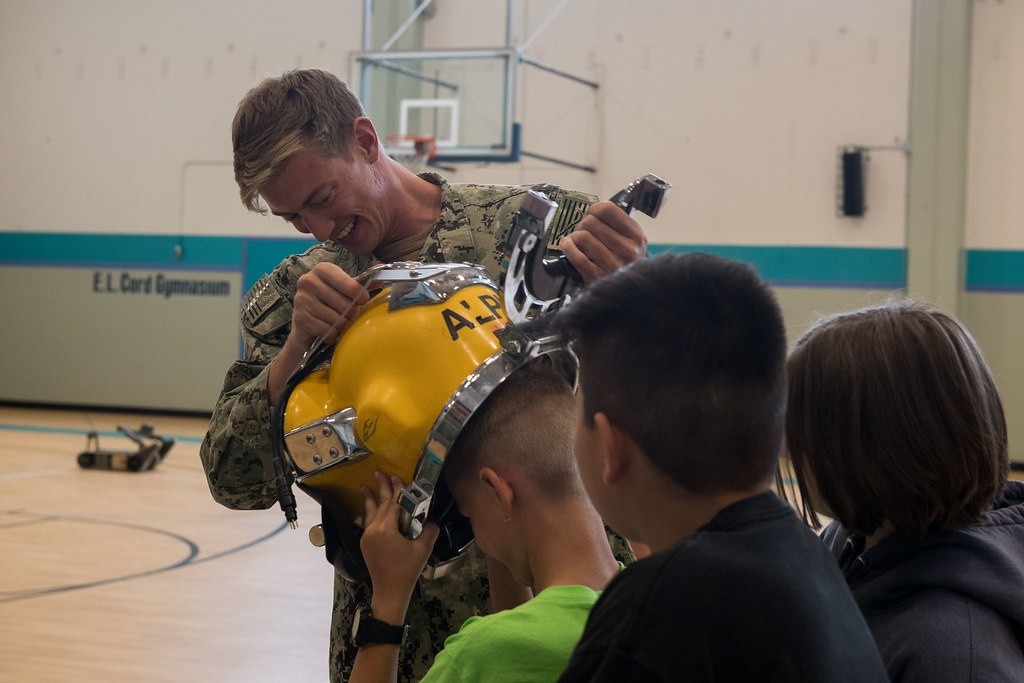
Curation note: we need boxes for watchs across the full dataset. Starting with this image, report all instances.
[350,603,409,647]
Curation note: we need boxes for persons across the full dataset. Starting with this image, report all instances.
[348,367,628,683]
[631,301,1024,683]
[550,251,888,683]
[201,69,648,683]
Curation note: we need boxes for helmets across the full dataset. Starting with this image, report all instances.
[274,254,581,575]
